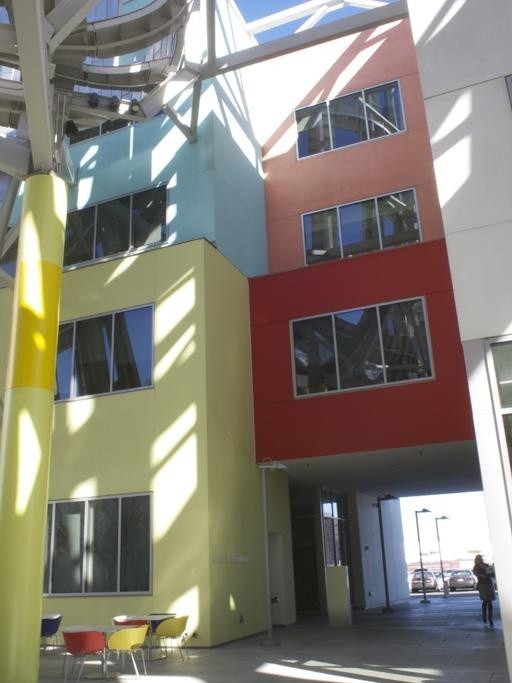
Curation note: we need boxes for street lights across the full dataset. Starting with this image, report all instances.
[435,515,449,597]
[415,508,431,602]
[376,495,398,612]
[257,459,287,647]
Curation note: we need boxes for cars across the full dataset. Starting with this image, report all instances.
[408,567,477,592]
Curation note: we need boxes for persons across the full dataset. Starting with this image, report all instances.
[472,552,497,627]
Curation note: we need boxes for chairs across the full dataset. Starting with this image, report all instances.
[40,614,188,683]
